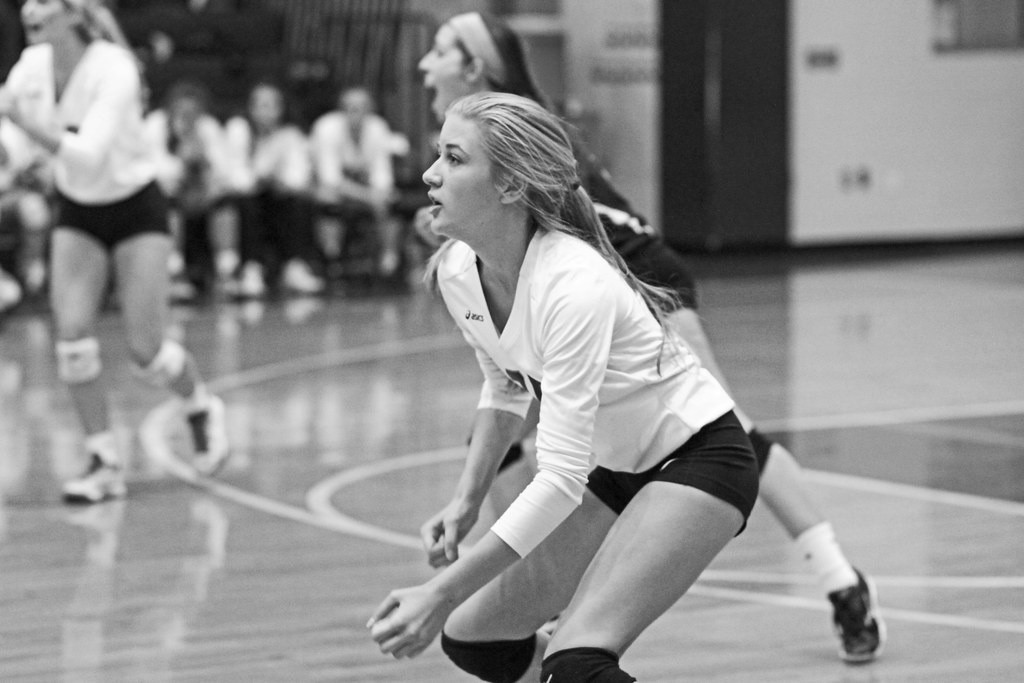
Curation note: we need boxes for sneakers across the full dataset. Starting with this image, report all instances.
[185,393,231,477]
[189,491,227,559]
[58,496,122,540]
[59,449,129,505]
[829,564,885,662]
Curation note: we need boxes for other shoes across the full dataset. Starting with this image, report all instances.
[215,265,242,302]
[237,258,266,299]
[166,274,199,305]
[280,258,326,295]
[326,263,346,297]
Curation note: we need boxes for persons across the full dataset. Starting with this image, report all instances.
[0,0,231,504]
[368,89,760,683]
[428,12,883,666]
[1,33,428,307]
